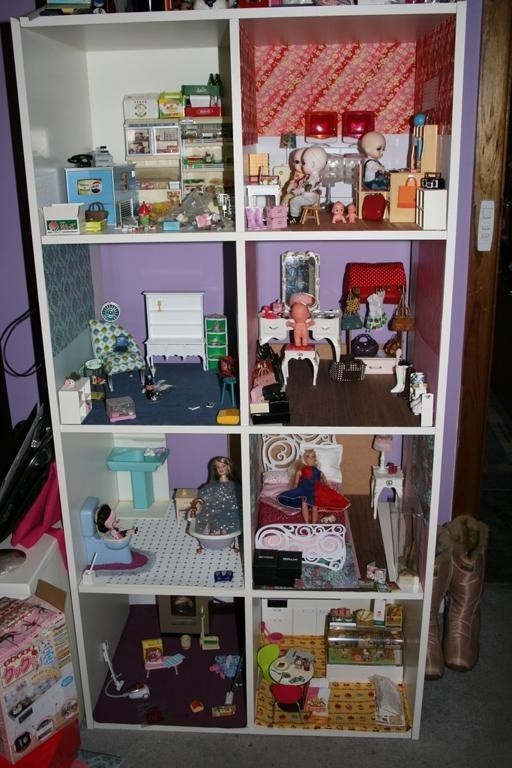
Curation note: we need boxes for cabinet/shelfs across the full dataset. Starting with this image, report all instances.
[8,1,484,742]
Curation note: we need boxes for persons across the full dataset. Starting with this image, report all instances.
[362,133,390,190]
[196,456,241,535]
[276,448,351,533]
[332,202,357,224]
[287,303,315,347]
[281,146,328,223]
[95,505,138,540]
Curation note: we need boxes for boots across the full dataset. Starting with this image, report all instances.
[443,515,492,674]
[424,523,455,684]
